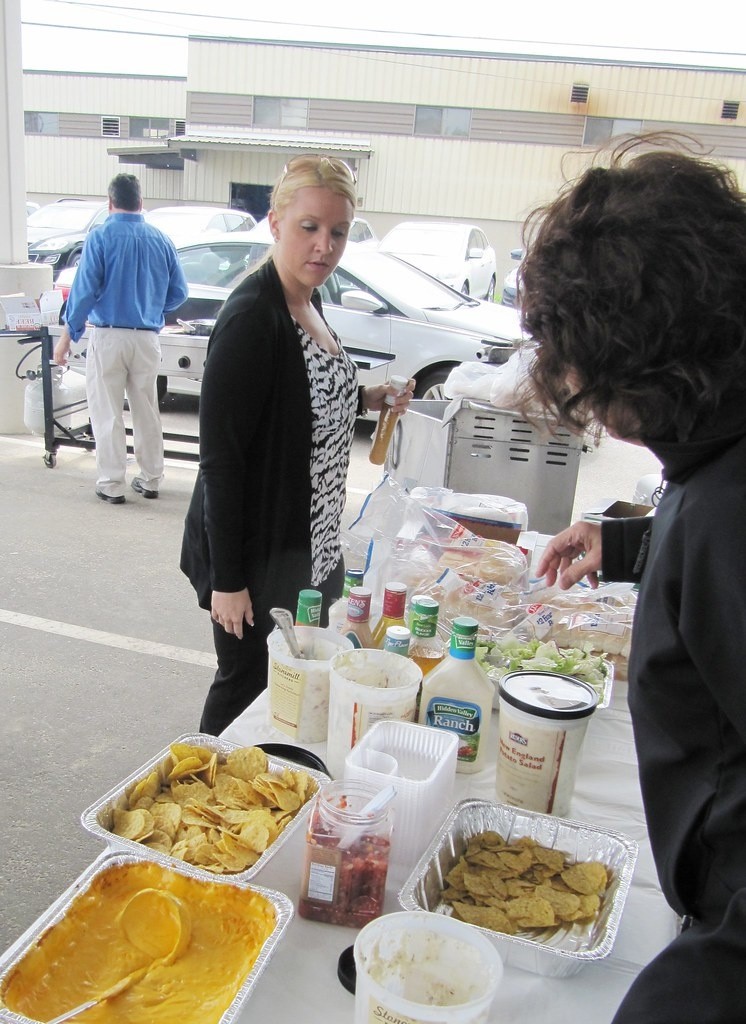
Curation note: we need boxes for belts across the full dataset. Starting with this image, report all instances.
[94,324,153,331]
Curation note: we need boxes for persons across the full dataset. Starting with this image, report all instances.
[180,157,417,737]
[515,131,746,1024]
[53,174,189,503]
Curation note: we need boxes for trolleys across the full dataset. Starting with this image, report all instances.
[0,325,199,469]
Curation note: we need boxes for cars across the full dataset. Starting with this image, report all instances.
[26,198,535,425]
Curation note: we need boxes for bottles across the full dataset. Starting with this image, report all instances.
[382,626,411,658]
[414,618,490,774]
[340,586,375,651]
[409,595,447,677]
[340,569,364,619]
[299,778,395,929]
[293,589,322,627]
[371,581,409,649]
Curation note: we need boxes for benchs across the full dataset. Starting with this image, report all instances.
[179,254,248,287]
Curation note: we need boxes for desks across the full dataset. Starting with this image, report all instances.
[217,530,681,1024]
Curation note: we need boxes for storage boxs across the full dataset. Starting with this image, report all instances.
[578,500,659,560]
[1,289,64,332]
[395,506,539,570]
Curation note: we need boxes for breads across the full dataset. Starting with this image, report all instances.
[412,544,635,658]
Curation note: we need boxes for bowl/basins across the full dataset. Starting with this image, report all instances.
[345,718,460,886]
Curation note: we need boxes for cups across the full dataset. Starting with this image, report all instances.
[498,669,600,820]
[353,908,503,1023]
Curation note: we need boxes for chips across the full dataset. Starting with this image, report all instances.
[111,741,316,875]
[440,830,610,936]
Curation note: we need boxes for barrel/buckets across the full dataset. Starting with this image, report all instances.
[266,625,355,744]
[327,646,424,771]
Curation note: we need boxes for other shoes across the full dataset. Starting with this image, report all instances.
[131,478,158,499]
[94,488,125,504]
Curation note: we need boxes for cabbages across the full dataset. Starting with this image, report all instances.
[472,639,610,706]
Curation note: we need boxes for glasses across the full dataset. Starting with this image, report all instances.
[273,153,358,212]
